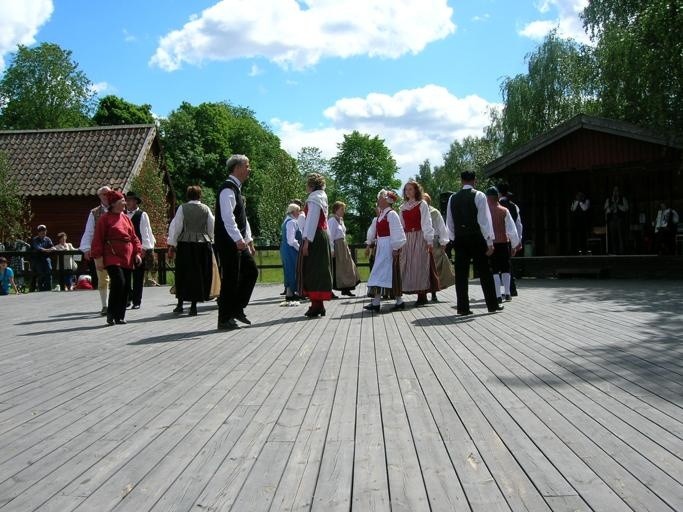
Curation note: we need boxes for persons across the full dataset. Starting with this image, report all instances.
[123,191,156,308]
[651,202,679,255]
[166,185,221,316]
[78,186,124,315]
[0,225,98,296]
[604,185,630,248]
[571,192,592,252]
[91,191,142,324]
[279,171,523,318]
[214,155,259,329]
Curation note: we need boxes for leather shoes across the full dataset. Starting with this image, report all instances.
[218,311,250,330]
[457,310,473,314]
[126,301,140,309]
[332,290,355,298]
[102,307,126,324]
[281,288,307,301]
[173,307,184,312]
[189,308,197,315]
[414,297,437,307]
[489,306,504,312]
[498,292,517,302]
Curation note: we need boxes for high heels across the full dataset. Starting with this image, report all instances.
[390,302,405,310]
[305,306,325,317]
[364,303,380,312]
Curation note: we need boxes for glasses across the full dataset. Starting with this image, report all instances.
[38,228,46,232]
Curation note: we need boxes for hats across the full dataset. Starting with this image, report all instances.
[37,224,46,229]
[108,191,124,205]
[126,191,141,204]
